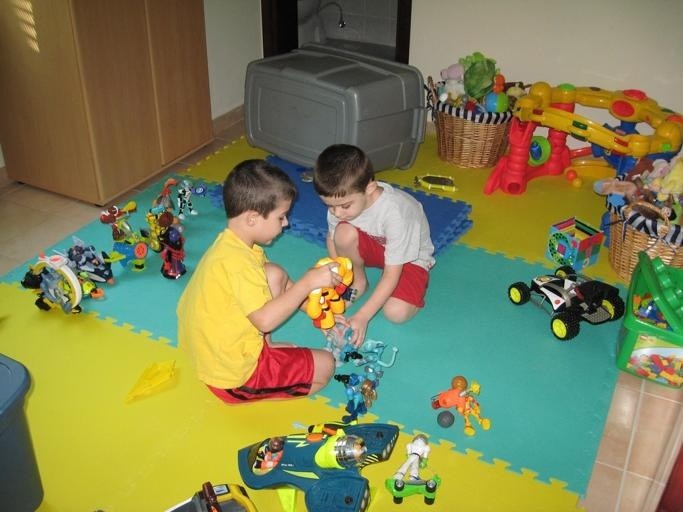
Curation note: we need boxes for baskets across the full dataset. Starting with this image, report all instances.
[427,76,510,169]
[608,202,683,285]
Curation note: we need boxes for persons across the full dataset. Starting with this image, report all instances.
[396,434,431,482]
[313,144,436,350]
[176,159,342,404]
[458,394,491,436]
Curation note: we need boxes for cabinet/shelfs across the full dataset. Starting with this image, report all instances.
[0,1,216,208]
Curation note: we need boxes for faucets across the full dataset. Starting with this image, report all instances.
[298,2,349,29]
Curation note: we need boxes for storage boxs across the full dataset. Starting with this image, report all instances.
[244,43,426,173]
[617,250,683,390]
